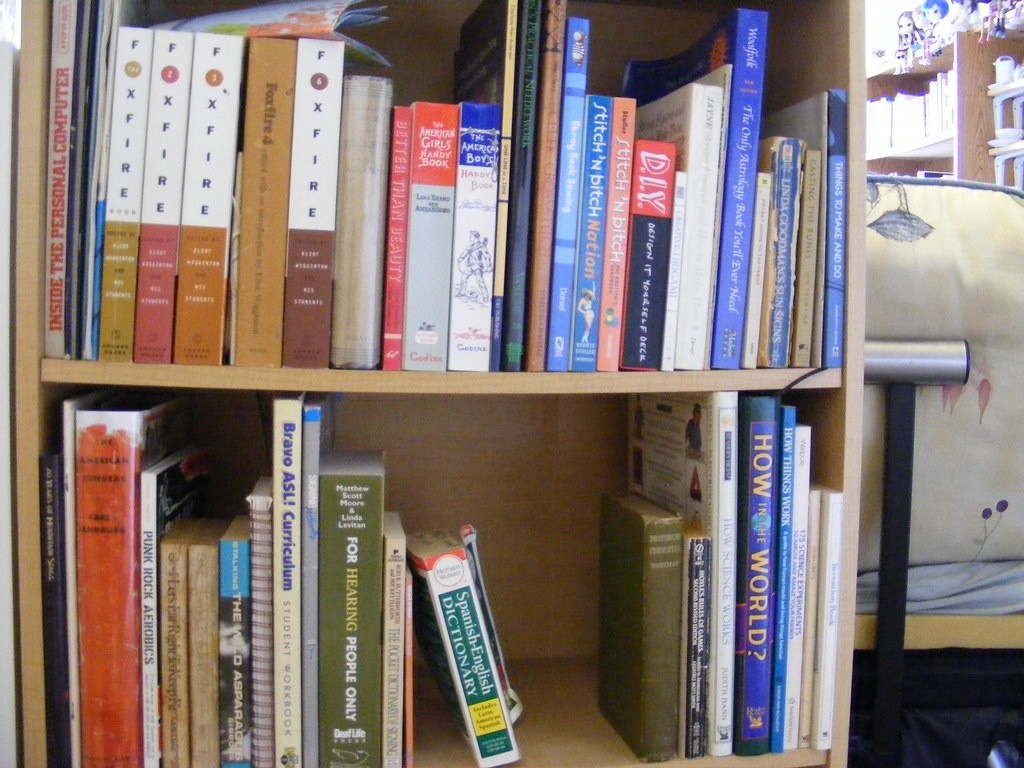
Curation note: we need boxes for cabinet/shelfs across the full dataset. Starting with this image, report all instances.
[2,0,1023,768]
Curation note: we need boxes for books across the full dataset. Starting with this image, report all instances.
[739,393,777,756]
[683,525,710,752]
[64,392,100,767]
[39,447,70,767]
[188,514,222,766]
[161,517,190,768]
[221,514,252,767]
[771,401,795,757]
[407,525,523,768]
[301,394,332,768]
[320,446,383,768]
[76,395,192,766]
[783,422,811,753]
[404,572,414,768]
[817,487,842,750]
[799,485,821,751]
[272,391,304,768]
[380,509,404,768]
[47,1,844,372]
[599,488,677,766]
[628,392,738,757]
[866,63,950,152]
[244,474,273,768]
[142,443,210,767]
